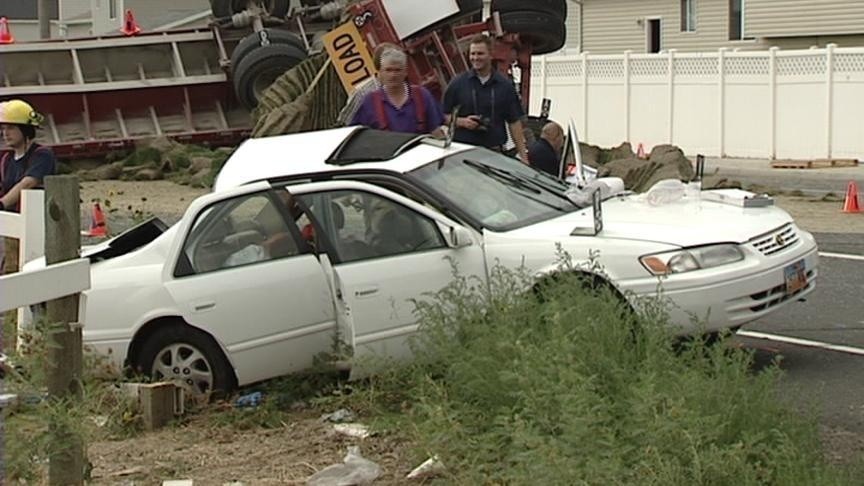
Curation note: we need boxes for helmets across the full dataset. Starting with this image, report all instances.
[0,98,45,127]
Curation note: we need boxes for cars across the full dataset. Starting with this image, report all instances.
[18,124,819,420]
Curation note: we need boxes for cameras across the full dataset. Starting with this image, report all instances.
[477,117,494,133]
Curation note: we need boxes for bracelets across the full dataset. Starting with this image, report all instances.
[0,201,5,210]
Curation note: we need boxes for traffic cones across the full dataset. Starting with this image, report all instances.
[636,142,645,159]
[86,203,109,236]
[0,17,14,44]
[839,182,859,213]
[120,9,141,36]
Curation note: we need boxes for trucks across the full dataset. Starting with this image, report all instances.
[1,0,567,180]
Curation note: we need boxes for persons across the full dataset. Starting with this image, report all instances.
[345,48,447,141]
[442,33,529,165]
[0,100,56,212]
[336,41,404,124]
[529,122,568,180]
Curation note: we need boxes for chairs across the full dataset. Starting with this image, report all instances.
[329,200,414,261]
